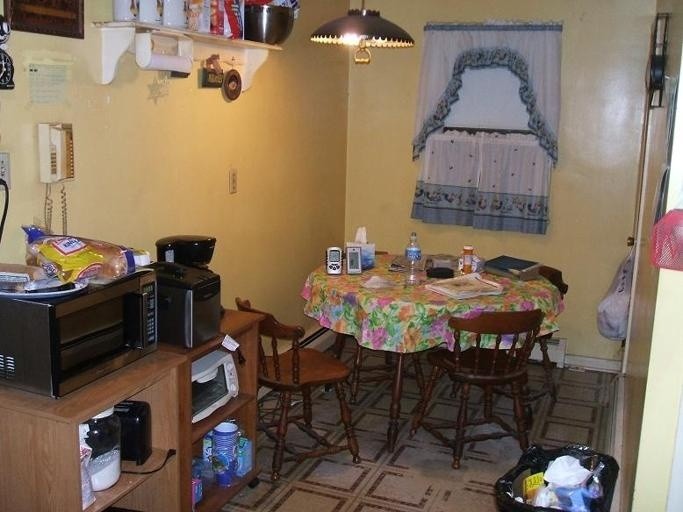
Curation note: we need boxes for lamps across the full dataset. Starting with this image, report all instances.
[307,0,415,63]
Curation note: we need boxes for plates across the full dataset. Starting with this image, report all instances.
[0,278,88,299]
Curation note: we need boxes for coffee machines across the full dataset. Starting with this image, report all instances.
[153,234,225,320]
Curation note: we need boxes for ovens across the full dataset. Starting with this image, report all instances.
[188,349,239,425]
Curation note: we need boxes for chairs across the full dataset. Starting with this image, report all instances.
[533,265,569,399]
[235,292,365,470]
[410,308,547,472]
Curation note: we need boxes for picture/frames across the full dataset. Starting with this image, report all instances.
[5,0,85,38]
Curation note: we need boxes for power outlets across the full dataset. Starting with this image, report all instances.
[1,152,10,190]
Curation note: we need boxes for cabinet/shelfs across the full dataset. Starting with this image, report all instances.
[1,310,266,511]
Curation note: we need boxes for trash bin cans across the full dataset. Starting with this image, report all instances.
[495,444,620,512]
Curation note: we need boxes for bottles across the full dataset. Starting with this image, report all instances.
[461,245,474,274]
[403,231,421,286]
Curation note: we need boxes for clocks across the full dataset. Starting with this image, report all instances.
[0,48,16,89]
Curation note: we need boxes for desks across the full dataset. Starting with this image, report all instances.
[301,252,568,453]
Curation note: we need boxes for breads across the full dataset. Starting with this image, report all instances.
[26,234,128,281]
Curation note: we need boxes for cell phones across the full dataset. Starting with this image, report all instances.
[346,247,362,275]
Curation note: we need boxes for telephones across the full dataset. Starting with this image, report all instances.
[38,123,74,184]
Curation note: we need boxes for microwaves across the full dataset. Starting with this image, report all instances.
[0,266,161,401]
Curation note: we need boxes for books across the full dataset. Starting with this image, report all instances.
[484,265,539,282]
[483,254,542,274]
